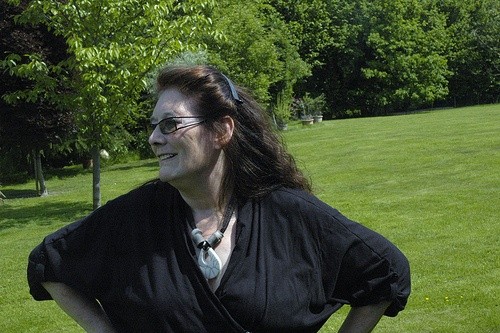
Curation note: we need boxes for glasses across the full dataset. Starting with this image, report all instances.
[144,116,209,136]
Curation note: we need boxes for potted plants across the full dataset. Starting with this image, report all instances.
[296,92,326,125]
[273,89,294,131]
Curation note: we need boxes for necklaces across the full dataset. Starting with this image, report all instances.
[175,183,238,280]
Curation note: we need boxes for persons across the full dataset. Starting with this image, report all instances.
[26,65,411,333]
